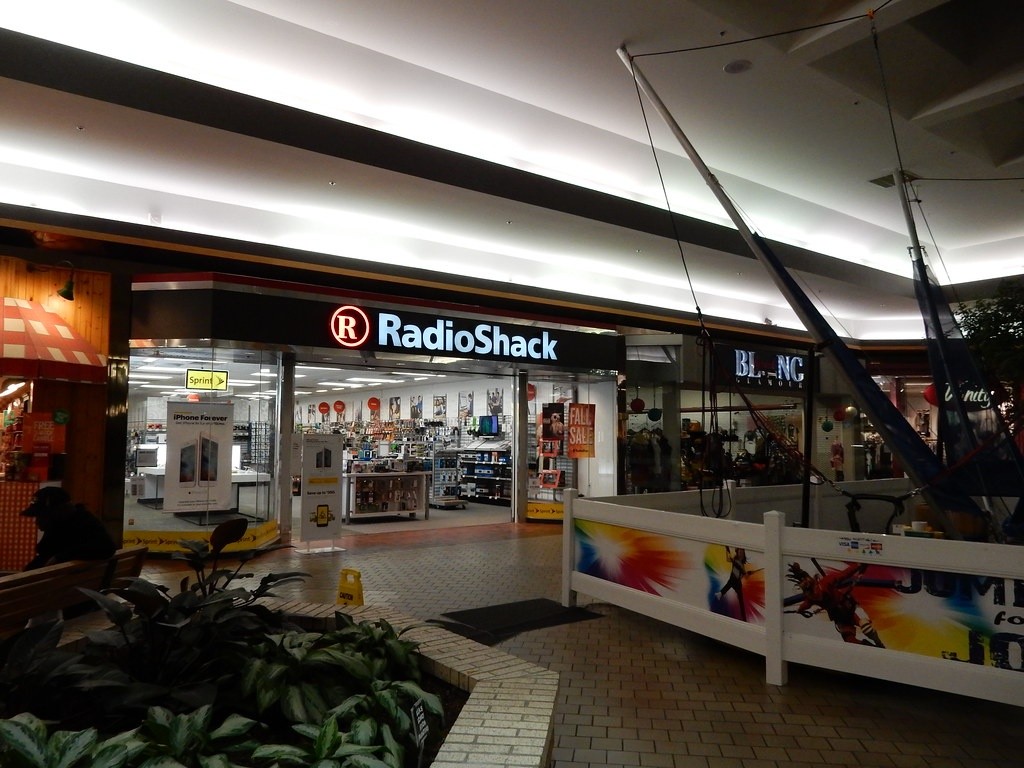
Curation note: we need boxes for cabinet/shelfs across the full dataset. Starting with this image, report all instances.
[292,414,580,513]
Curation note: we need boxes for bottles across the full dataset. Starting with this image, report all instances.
[0,409,32,483]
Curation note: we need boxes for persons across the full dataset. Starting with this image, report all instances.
[433,399,442,416]
[15,484,120,624]
[411,397,417,409]
[492,392,503,412]
[467,393,473,415]
[413,395,422,418]
[488,391,499,408]
[439,397,447,416]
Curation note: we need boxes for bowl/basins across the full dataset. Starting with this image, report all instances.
[911,521,927,531]
